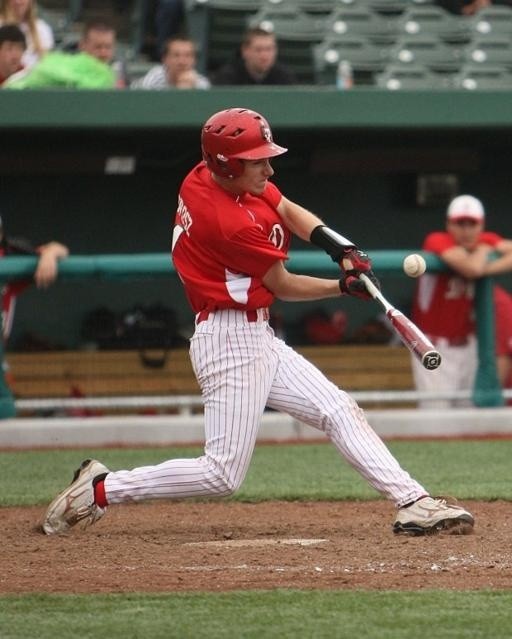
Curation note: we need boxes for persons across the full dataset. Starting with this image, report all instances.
[0,24,34,92]
[128,33,212,91]
[209,26,299,86]
[406,191,511,408]
[75,16,128,91]
[40,104,476,539]
[1,1,56,71]
[0,229,72,402]
[492,282,512,408]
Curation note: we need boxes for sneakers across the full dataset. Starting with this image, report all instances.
[39,457,111,542]
[393,496,475,537]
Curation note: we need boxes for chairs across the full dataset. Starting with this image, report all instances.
[247,2,512,97]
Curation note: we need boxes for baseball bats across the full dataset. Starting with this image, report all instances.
[356,271,441,370]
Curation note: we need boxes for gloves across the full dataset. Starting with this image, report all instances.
[338,246,372,278]
[339,271,381,302]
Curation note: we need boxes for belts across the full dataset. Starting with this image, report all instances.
[197,310,258,324]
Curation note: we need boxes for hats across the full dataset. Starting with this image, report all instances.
[446,194,484,223]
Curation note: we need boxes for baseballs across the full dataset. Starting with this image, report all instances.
[403,253,426,278]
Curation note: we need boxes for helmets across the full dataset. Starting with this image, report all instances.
[200,107,289,182]
